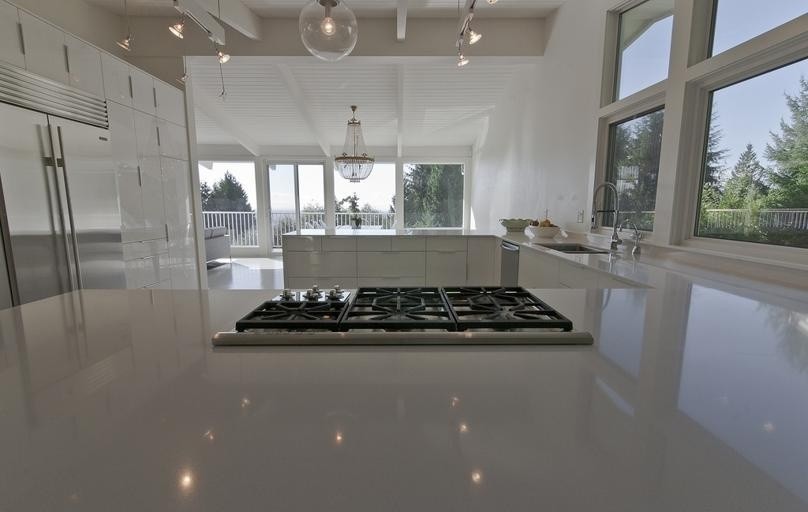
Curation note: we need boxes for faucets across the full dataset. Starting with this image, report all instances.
[589,181,622,249]
[618,216,644,256]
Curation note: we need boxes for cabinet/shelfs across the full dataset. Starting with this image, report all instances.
[283,236,356,289]
[356,236,427,287]
[160,156,200,290]
[0,0,65,83]
[427,236,497,286]
[64,31,132,107]
[518,246,643,291]
[156,116,188,162]
[105,98,165,243]
[132,63,186,125]
[123,238,171,291]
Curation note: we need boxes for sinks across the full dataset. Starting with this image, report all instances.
[532,241,608,255]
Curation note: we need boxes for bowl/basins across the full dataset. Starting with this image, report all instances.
[499,218,530,234]
[527,225,559,238]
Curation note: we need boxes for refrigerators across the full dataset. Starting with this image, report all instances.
[0,59,128,308]
[12,290,134,412]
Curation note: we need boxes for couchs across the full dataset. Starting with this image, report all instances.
[205,225,232,264]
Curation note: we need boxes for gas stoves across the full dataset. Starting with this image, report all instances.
[212,281,596,349]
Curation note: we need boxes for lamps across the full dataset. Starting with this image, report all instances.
[111,1,136,52]
[167,1,230,63]
[297,0,359,62]
[454,0,501,69]
[333,104,375,184]
